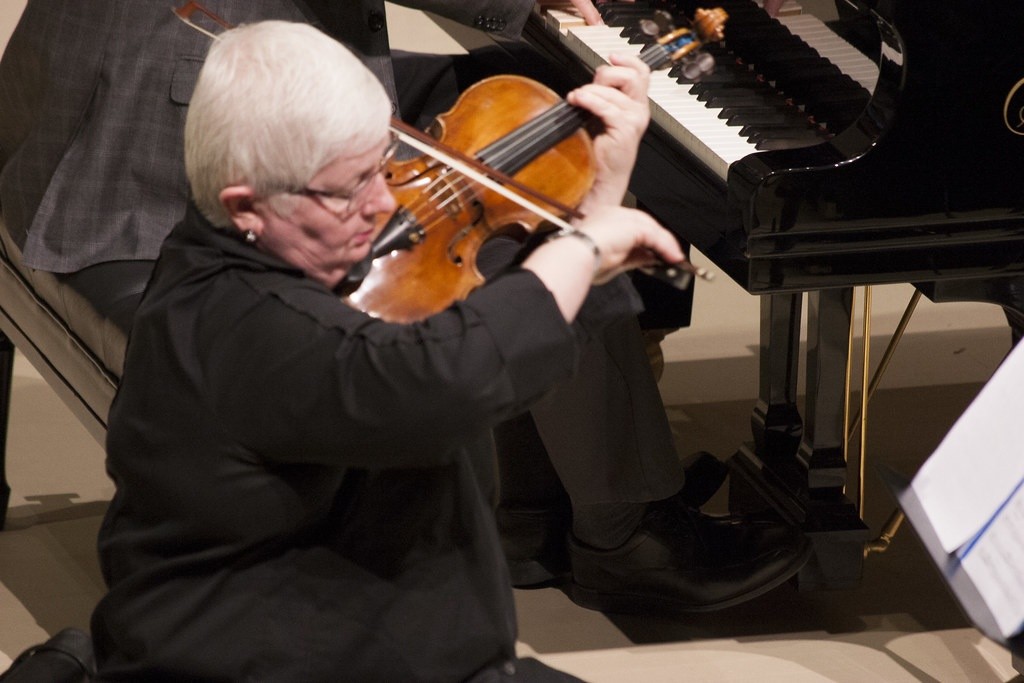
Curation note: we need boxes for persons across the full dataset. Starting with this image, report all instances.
[88,17,689,683]
[0,0,815,617]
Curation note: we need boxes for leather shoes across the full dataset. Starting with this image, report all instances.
[495,451,728,586]
[566,501,814,611]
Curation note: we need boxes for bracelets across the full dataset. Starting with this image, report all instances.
[545,226,605,279]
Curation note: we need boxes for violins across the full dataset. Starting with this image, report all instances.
[336,6,729,326]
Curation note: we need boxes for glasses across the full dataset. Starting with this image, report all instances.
[269,127,399,214]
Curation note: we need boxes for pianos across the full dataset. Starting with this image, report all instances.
[518,0,1024,529]
